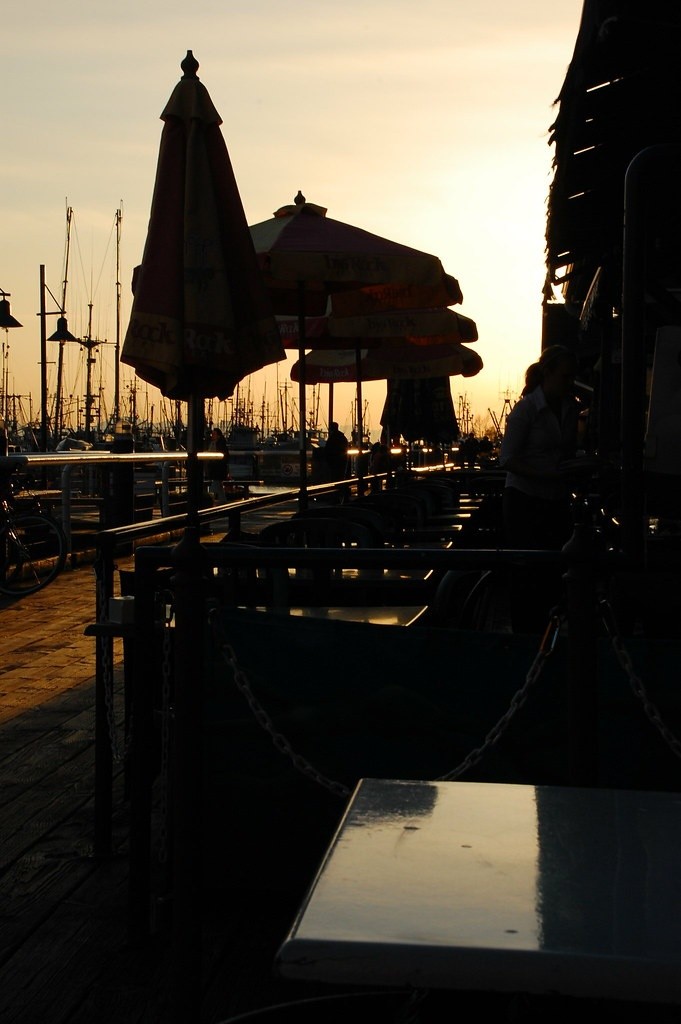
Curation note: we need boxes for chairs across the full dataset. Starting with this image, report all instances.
[253,473,459,605]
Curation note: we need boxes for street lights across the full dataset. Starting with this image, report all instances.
[34,263,83,489]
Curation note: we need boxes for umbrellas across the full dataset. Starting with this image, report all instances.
[277,308,481,496]
[132,191,461,510]
[121,50,286,1023]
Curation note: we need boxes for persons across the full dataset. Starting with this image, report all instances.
[372,442,388,471]
[499,345,589,634]
[205,428,230,503]
[351,429,357,444]
[316,422,347,481]
[463,433,493,469]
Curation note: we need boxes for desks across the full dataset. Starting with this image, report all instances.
[272,778,681,1004]
[239,494,490,628]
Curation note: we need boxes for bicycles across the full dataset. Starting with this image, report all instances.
[0,465,70,597]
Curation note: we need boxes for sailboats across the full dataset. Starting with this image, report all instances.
[1,192,374,500]
